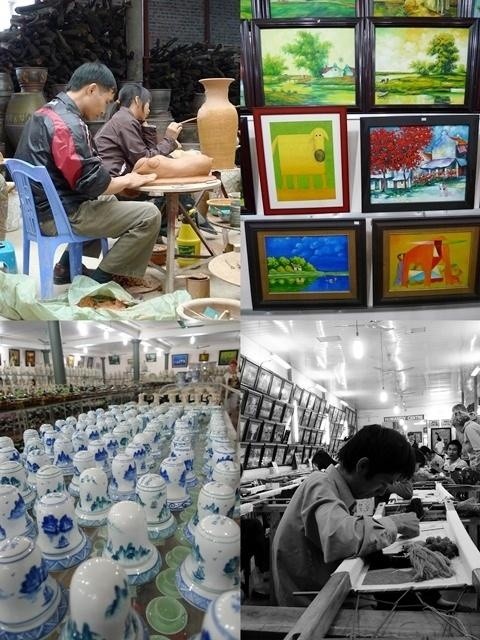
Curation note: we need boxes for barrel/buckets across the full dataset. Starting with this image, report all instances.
[208,251,242,301]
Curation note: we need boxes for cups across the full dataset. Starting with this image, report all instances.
[0,401,240,640]
[186,274,211,299]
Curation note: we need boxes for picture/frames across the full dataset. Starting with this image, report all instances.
[8,349,35,366]
[431,427,452,453]
[172,349,239,368]
[239,353,358,470]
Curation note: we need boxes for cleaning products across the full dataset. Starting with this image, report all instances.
[176,209,202,268]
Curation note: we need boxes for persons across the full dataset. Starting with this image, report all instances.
[239,432,480,600]
[13,61,162,294]
[269,421,421,609]
[451,410,480,476]
[451,404,480,467]
[91,82,196,245]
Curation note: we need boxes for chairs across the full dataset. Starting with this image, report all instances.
[3,158,109,300]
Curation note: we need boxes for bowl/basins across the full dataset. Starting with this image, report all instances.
[148,244,167,268]
[217,209,231,223]
[206,198,233,217]
[176,297,240,321]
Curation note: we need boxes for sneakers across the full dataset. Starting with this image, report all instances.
[183,219,218,235]
[436,597,459,610]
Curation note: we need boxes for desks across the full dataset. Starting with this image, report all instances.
[130,175,222,294]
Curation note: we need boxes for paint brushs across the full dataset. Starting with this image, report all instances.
[181,117,197,124]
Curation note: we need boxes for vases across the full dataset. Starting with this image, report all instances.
[0,67,68,151]
[147,89,173,115]
[196,77,239,169]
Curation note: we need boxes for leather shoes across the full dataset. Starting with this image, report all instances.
[53,264,96,285]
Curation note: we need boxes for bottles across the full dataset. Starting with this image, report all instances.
[230,199,241,227]
